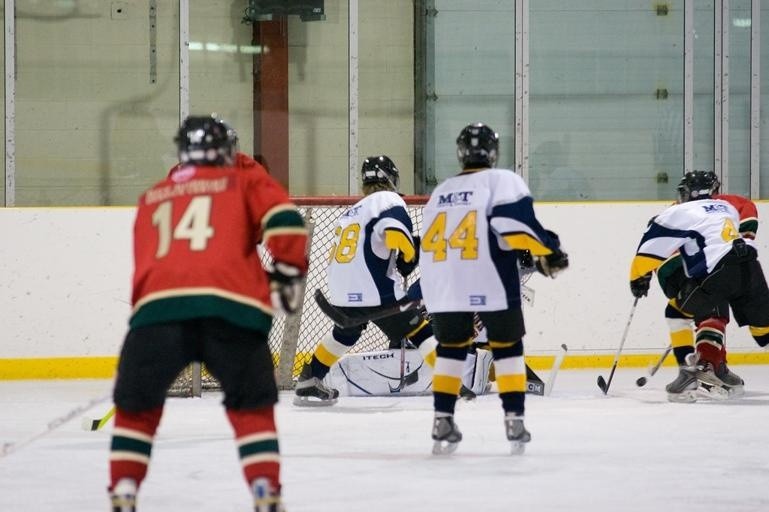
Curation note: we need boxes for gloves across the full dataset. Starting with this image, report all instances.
[631,277,650,298]
[396,251,415,275]
[536,251,569,277]
[278,262,301,312]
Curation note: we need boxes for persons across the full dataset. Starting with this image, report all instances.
[418,122,569,455]
[293,154,491,408]
[108,112,308,512]
[668,171,758,402]
[629,170,768,404]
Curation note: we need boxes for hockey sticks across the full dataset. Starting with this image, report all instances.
[82,404,117,432]
[313,251,568,329]
[598,295,640,395]
[391,273,410,393]
[635,344,674,387]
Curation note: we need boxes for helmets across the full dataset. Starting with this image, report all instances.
[677,170,714,204]
[457,121,499,166]
[708,171,720,193]
[362,154,400,191]
[177,113,238,164]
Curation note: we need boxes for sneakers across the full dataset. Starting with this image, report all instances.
[295,376,339,400]
[431,415,463,442]
[694,359,744,387]
[113,502,135,512]
[667,368,699,393]
[459,386,476,399]
[504,417,531,442]
[257,499,278,512]
